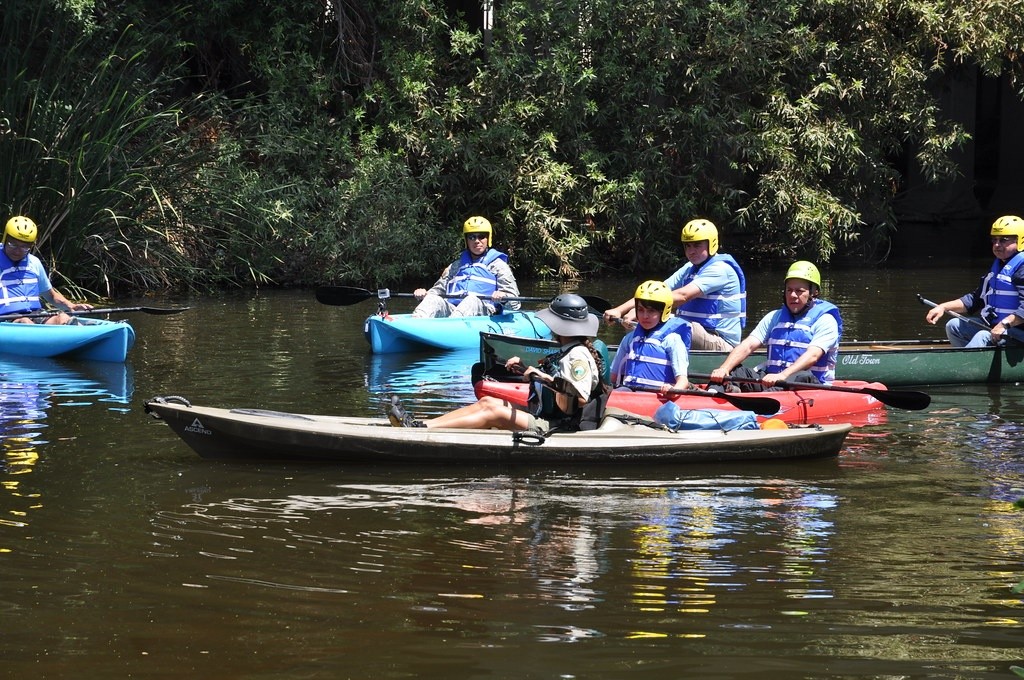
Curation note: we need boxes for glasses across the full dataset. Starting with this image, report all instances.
[991,237,1010,243]
[6,240,31,251]
[468,234,485,240]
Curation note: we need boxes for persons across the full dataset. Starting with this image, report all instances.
[610,281,692,399]
[387,294,611,431]
[603,219,746,350]
[413,216,521,316]
[0,216,93,326]
[707,261,842,393]
[926,216,1024,346]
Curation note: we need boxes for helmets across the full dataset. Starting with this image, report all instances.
[2,216,37,254]
[535,294,600,337]
[635,280,673,323]
[785,261,821,290]
[681,219,719,256]
[990,215,1024,252]
[462,216,493,247]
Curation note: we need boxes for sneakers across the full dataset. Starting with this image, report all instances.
[387,395,427,428]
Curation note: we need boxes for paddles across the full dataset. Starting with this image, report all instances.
[481,335,579,398]
[0,306,191,322]
[315,285,612,314]
[687,374,931,411]
[917,293,1024,349]
[622,381,781,416]
[589,311,619,322]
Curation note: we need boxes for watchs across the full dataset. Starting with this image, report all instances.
[1002,320,1011,329]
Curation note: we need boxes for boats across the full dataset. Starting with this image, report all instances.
[365,310,552,354]
[145,397,852,467]
[478,331,1024,387]
[475,377,889,422]
[0,318,135,362]
